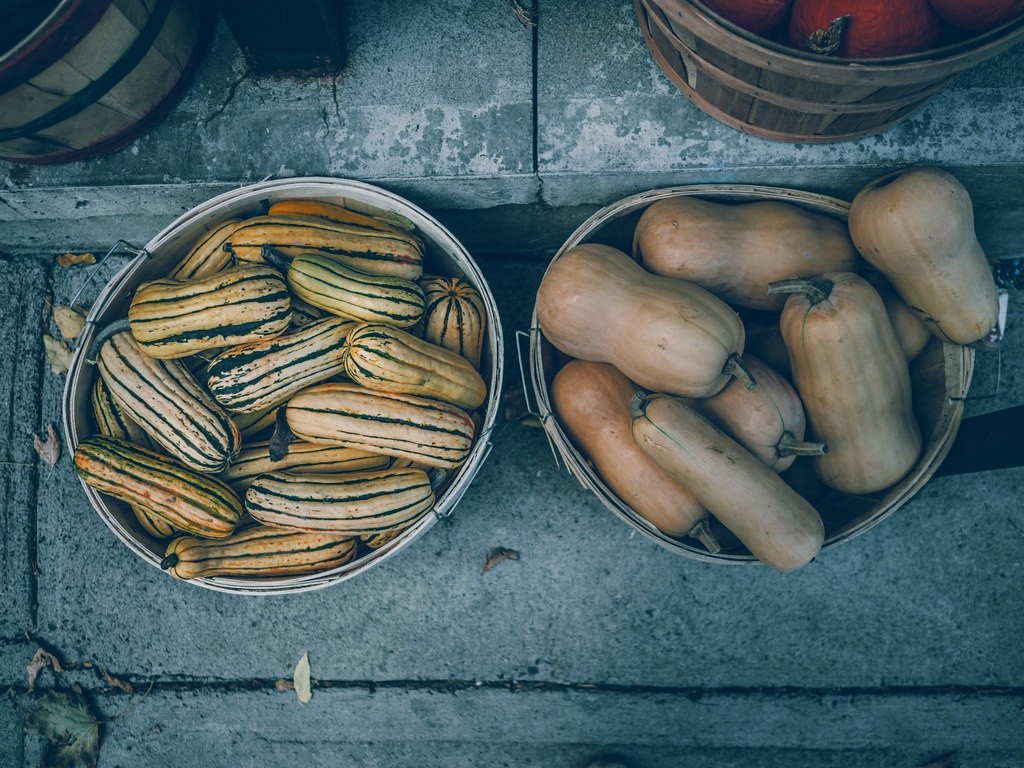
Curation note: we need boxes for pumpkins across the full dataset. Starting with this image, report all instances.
[534,164,1000,573]
[698,0,1024,59]
[72,201,486,579]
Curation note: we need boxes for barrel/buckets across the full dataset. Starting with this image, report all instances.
[62,178,503,595]
[0,0,215,163]
[634,0,1024,143]
[518,185,1001,569]
